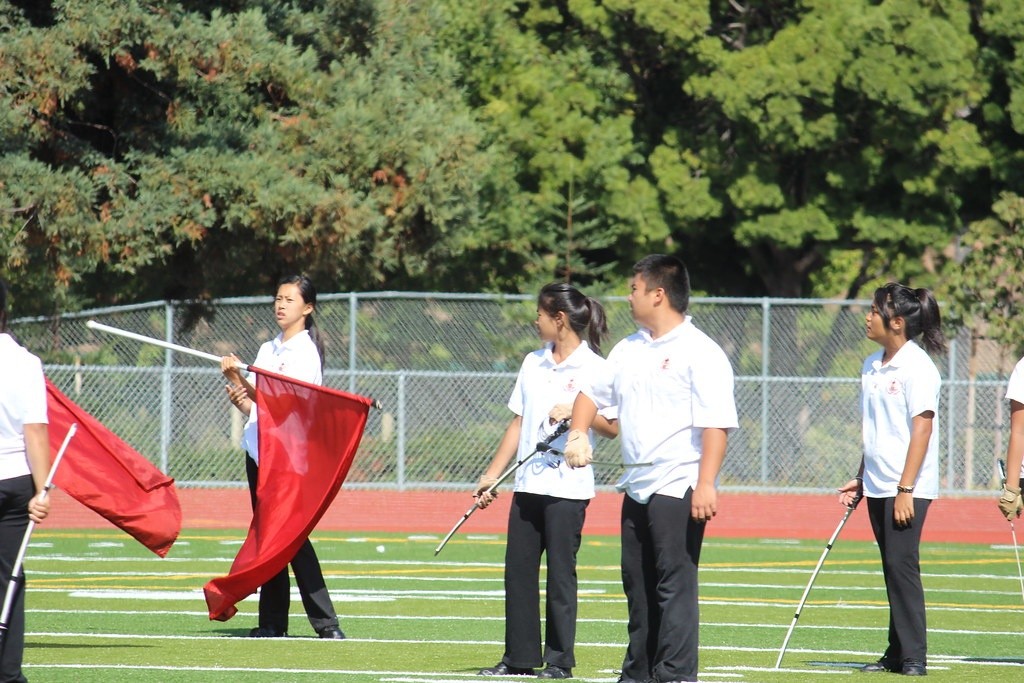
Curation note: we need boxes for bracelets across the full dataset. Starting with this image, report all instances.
[851,477,862,481]
[897,484,914,493]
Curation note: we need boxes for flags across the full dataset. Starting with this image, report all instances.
[42,370,183,559]
[202,365,371,622]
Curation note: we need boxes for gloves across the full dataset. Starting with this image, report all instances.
[563,430,592,469]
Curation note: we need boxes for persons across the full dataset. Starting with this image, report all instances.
[473,281,618,678]
[998,355,1024,521]
[0,278,50,683]
[838,281,942,675]
[562,253,740,683]
[220,270,349,639]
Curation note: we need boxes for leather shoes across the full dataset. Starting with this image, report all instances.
[478,662,535,675]
[903,659,927,676]
[537,663,573,679]
[863,661,891,672]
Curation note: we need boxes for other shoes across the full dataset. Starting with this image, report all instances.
[250,627,287,637]
[319,628,345,639]
